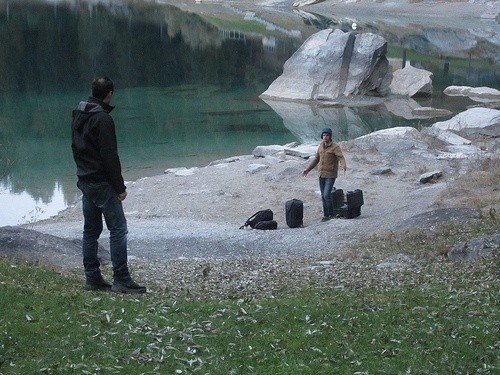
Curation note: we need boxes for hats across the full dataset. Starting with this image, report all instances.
[321,128,332,139]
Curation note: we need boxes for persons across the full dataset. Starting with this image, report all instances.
[71,76,147,294]
[301,127,346,222]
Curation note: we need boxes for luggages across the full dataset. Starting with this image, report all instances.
[340,189,363,219]
[286,199,303,228]
[329,187,344,219]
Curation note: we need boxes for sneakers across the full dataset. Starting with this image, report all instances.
[111,277,146,294]
[85,277,112,290]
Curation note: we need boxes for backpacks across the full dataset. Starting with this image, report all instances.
[239,209,273,229]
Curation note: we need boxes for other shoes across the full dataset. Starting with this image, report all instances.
[320,215,336,222]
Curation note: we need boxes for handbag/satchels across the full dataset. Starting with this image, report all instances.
[254,220,277,230]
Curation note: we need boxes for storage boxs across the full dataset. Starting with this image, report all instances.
[285,199,303,228]
[340,189,364,219]
[331,187,344,218]
